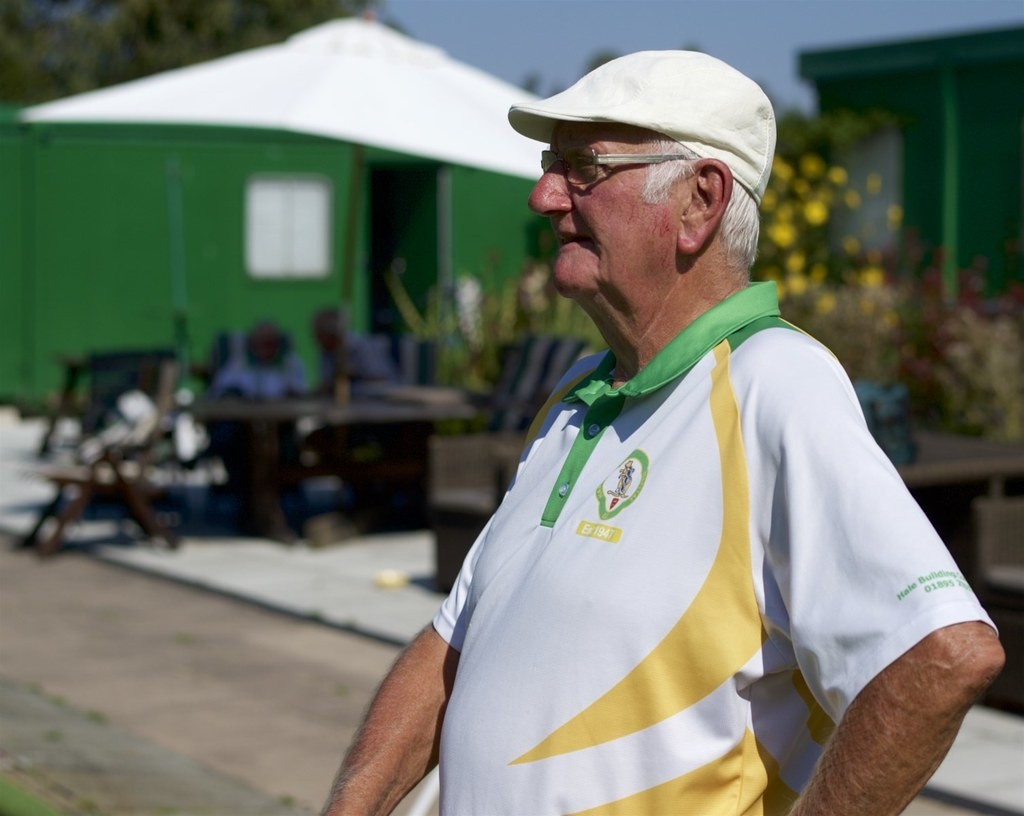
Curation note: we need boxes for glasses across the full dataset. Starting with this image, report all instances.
[541,149,681,185]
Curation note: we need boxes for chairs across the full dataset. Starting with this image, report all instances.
[21,348,174,555]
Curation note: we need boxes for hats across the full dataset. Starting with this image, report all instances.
[506,50,777,208]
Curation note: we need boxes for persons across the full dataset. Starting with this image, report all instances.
[321,49,1006,815]
[202,307,392,532]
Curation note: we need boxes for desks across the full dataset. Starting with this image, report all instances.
[869,425,1024,595]
[182,399,476,540]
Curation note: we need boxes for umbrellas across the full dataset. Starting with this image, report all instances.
[15,12,552,406]
